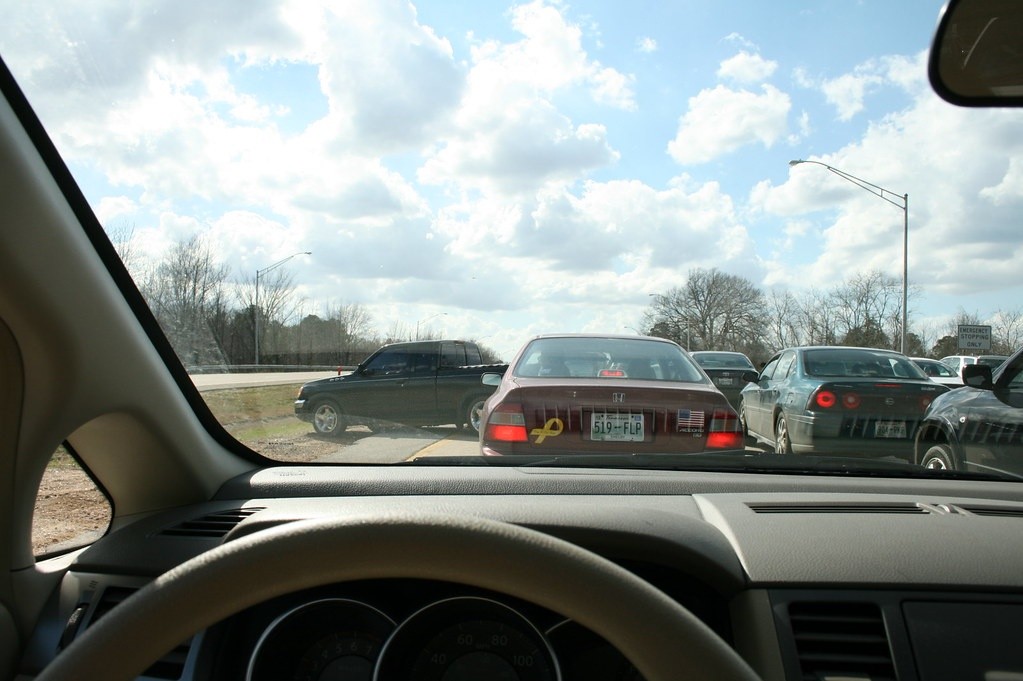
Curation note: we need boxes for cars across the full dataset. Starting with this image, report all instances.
[681,350,760,411]
[937,353,1011,383]
[293,339,509,439]
[738,345,958,468]
[477,331,744,464]
[911,343,1023,484]
[886,356,965,387]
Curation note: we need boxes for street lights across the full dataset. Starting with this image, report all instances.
[416,312,447,342]
[789,158,908,357]
[649,294,690,353]
[254,252,314,372]
